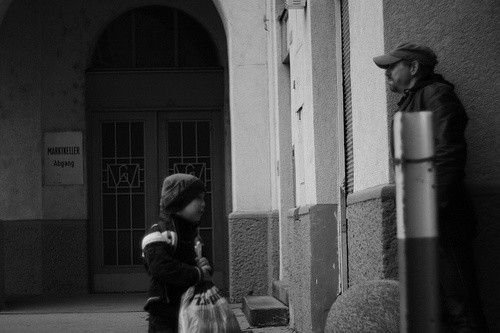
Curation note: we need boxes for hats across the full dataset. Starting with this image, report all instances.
[160,173,205,212]
[373,41,438,69]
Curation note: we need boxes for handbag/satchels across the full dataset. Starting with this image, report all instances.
[179,280,242,333]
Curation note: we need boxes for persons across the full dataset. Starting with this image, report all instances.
[373,42,470,238]
[140,174,211,333]
